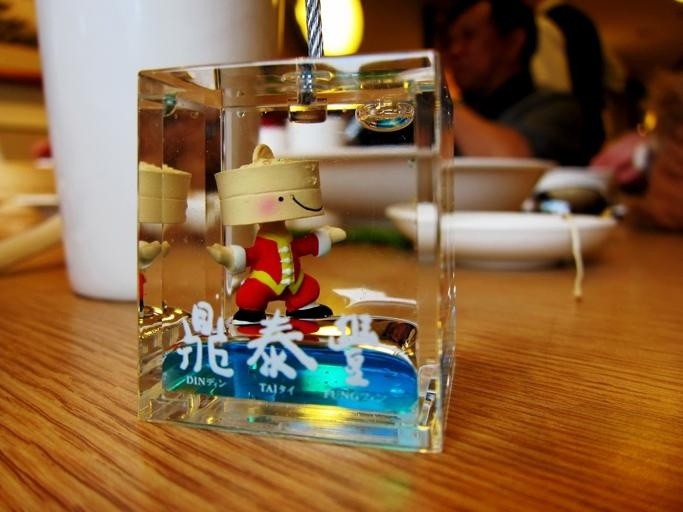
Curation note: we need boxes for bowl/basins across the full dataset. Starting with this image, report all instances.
[285,148,552,211]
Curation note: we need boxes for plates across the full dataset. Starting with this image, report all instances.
[387,206,619,271]
[383,204,439,259]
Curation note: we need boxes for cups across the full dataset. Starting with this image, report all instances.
[36,0,284,303]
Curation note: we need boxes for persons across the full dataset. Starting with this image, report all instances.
[419,0,604,218]
[590,67,682,235]
